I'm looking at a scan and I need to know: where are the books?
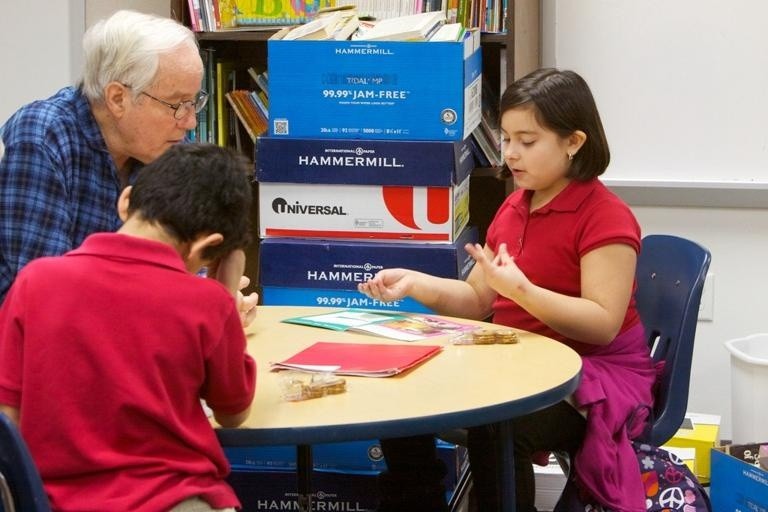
[267,340,445,379]
[187,1,507,169]
[279,306,484,344]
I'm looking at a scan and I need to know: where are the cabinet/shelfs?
[170,1,541,305]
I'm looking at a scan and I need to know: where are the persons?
[0,139,264,512]
[354,66,659,511]
[0,8,206,302]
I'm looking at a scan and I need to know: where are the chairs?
[550,234,710,447]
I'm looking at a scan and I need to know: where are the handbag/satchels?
[585,440,713,512]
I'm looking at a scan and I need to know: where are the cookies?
[301,379,345,397]
[472,328,516,344]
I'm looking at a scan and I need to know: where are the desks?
[199,304,582,512]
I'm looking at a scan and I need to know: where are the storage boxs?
[708,442,766,510]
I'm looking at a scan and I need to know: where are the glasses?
[123,84,209,120]
